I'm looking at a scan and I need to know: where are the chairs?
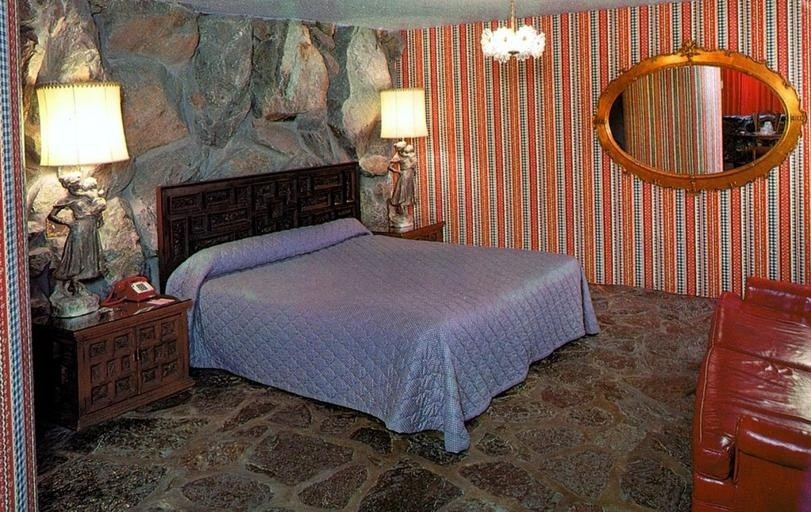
[735,114,776,161]
[763,114,787,143]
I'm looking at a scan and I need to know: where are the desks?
[375,221,447,242]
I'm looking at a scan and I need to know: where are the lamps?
[381,88,430,230]
[480,0,546,66]
[38,84,131,317]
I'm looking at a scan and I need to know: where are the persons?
[69,176,107,229]
[45,170,109,295]
[397,145,417,208]
[387,141,415,216]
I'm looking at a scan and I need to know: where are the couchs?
[692,274,811,512]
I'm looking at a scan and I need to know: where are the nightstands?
[33,297,193,432]
[156,161,600,452]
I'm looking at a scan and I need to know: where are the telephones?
[115,276,156,302]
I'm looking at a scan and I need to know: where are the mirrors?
[593,39,808,197]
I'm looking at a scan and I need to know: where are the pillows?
[198,218,364,276]
